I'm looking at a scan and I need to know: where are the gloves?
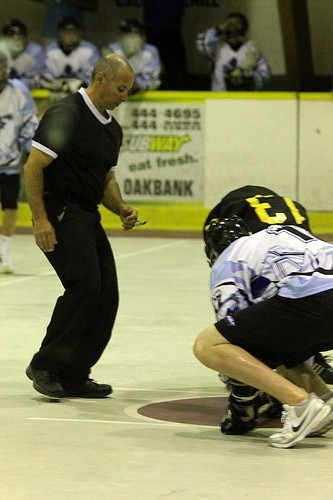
[220,392,284,435]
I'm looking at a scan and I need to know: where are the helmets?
[120,18,142,35]
[202,215,250,267]
[3,19,27,38]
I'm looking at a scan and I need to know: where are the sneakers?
[302,352,333,384]
[267,392,333,448]
[0,253,13,274]
[65,377,111,397]
[26,359,65,398]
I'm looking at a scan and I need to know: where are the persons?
[23,54,137,400]
[1,51,42,277]
[194,185,333,448]
[2,13,271,95]
